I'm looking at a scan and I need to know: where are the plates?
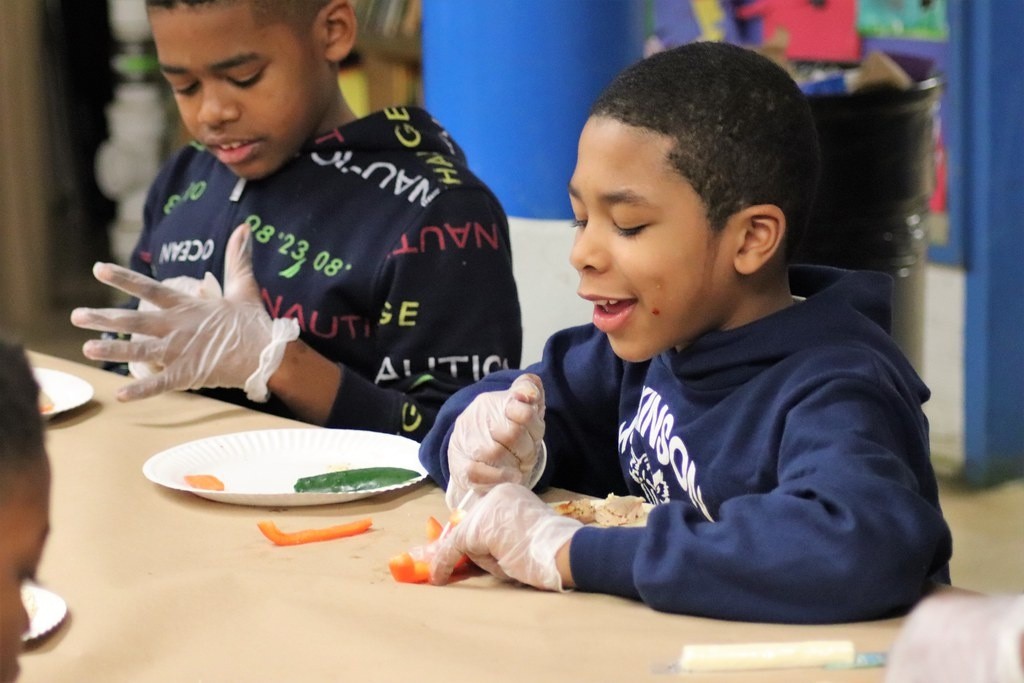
[139,427,431,509]
[14,582,69,644]
[30,368,99,422]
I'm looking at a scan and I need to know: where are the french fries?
[257,520,371,544]
[389,519,469,583]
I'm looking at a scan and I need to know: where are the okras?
[293,467,420,493]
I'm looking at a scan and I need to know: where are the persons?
[0,344,50,683]
[419,41,953,624]
[71,0,522,442]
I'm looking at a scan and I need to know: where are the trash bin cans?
[786,56,944,395]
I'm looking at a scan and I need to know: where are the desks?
[17,347,989,682]
